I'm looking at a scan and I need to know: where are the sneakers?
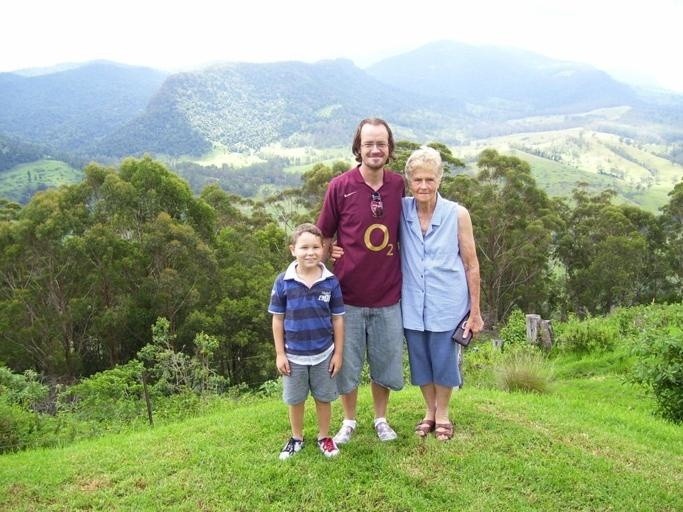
[317,425,355,458]
[373,421,397,442]
[278,437,305,461]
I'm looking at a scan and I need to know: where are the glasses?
[360,142,390,150]
[370,190,384,217]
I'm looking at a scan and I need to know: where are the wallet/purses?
[452,310,474,348]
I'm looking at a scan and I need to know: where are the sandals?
[414,416,455,441]
